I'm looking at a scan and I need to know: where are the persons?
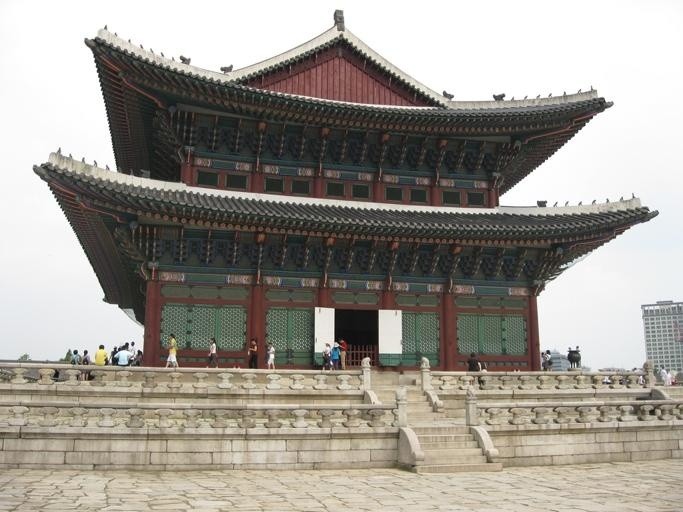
[338,337,348,370]
[265,342,276,369]
[541,352,547,361]
[329,342,340,370]
[466,351,479,371]
[321,343,332,370]
[245,339,259,368]
[164,333,180,369]
[601,366,678,386]
[67,341,144,381]
[206,337,219,368]
[541,349,552,371]
